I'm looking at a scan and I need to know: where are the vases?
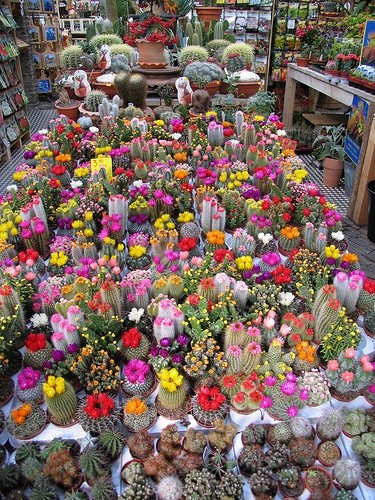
[295,57,311,67]
[92,81,118,99]
[195,7,223,29]
[326,70,339,77]
[134,39,164,64]
[232,81,263,99]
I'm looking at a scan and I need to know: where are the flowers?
[0,0,375,425]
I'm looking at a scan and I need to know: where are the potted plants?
[312,123,346,187]
[80,53,102,83]
[78,88,109,118]
[183,61,223,97]
[55,81,81,122]
[188,90,213,118]
[0,406,375,500]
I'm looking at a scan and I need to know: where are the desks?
[282,62,375,225]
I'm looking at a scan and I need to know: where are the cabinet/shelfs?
[0,0,32,168]
[25,0,65,101]
